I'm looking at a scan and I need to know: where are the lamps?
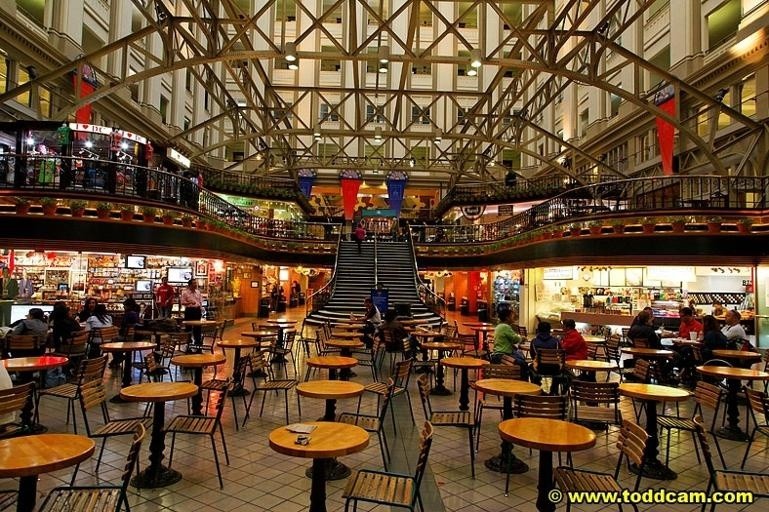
[379,65,388,73]
[288,54,299,70]
[285,42,296,62]
[374,126,383,139]
[379,46,389,64]
[471,49,482,68]
[313,124,321,137]
[466,58,477,76]
[434,128,441,141]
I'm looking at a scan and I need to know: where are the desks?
[260,323,293,364]
[325,339,364,378]
[217,338,259,397]
[136,328,191,350]
[421,341,462,395]
[183,319,217,351]
[440,357,490,427]
[660,337,690,382]
[267,319,297,353]
[100,340,157,404]
[295,380,364,481]
[498,417,597,512]
[0,357,68,435]
[332,332,364,359]
[120,382,199,491]
[618,383,689,481]
[461,322,493,355]
[241,330,278,378]
[306,357,359,423]
[335,323,364,332]
[469,326,495,361]
[620,346,673,403]
[696,365,768,441]
[168,353,226,416]
[0,433,96,512]
[269,421,369,512]
[566,359,618,431]
[712,348,761,405]
[410,331,447,375]
[475,378,541,474]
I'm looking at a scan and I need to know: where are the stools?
[699,469,769,512]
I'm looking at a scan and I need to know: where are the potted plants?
[552,224,563,238]
[570,223,582,236]
[706,218,722,231]
[588,221,602,234]
[671,218,686,231]
[40,197,58,215]
[69,199,88,218]
[161,210,177,225]
[13,195,30,215]
[195,216,205,229]
[119,206,135,221]
[612,221,625,234]
[140,206,157,223]
[95,201,112,218]
[181,214,192,227]
[736,218,754,234]
[640,218,657,233]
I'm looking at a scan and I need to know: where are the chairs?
[32,353,108,434]
[415,372,476,477]
[139,338,176,383]
[454,320,475,356]
[338,377,395,471]
[402,337,436,389]
[216,320,236,357]
[380,327,406,376]
[456,326,481,358]
[88,326,111,358]
[4,334,39,358]
[622,359,649,422]
[502,393,573,498]
[197,354,250,431]
[605,343,621,382]
[355,357,415,433]
[474,364,519,452]
[603,334,630,383]
[52,331,89,378]
[461,337,483,381]
[312,328,341,376]
[68,378,154,495]
[528,348,566,395]
[470,326,495,355]
[164,388,230,489]
[341,420,434,511]
[634,338,662,384]
[99,327,125,381]
[622,328,634,350]
[354,336,382,382]
[690,342,718,385]
[242,352,302,428]
[0,381,35,442]
[657,381,727,471]
[38,423,145,512]
[580,333,608,360]
[552,419,650,512]
[587,344,607,363]
[565,379,633,470]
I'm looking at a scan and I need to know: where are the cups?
[689,332,697,340]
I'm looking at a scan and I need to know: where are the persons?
[108,298,139,369]
[13,308,49,358]
[355,224,364,253]
[352,297,381,349]
[494,309,530,382]
[644,306,654,321]
[628,311,680,385]
[557,319,587,395]
[700,315,728,383]
[1,360,17,425]
[50,301,81,363]
[180,279,202,354]
[379,309,422,353]
[293,280,301,306]
[179,169,203,212]
[86,304,113,358]
[713,303,728,317]
[675,308,704,337]
[80,297,97,323]
[721,310,748,339]
[530,322,561,395]
[156,277,175,317]
